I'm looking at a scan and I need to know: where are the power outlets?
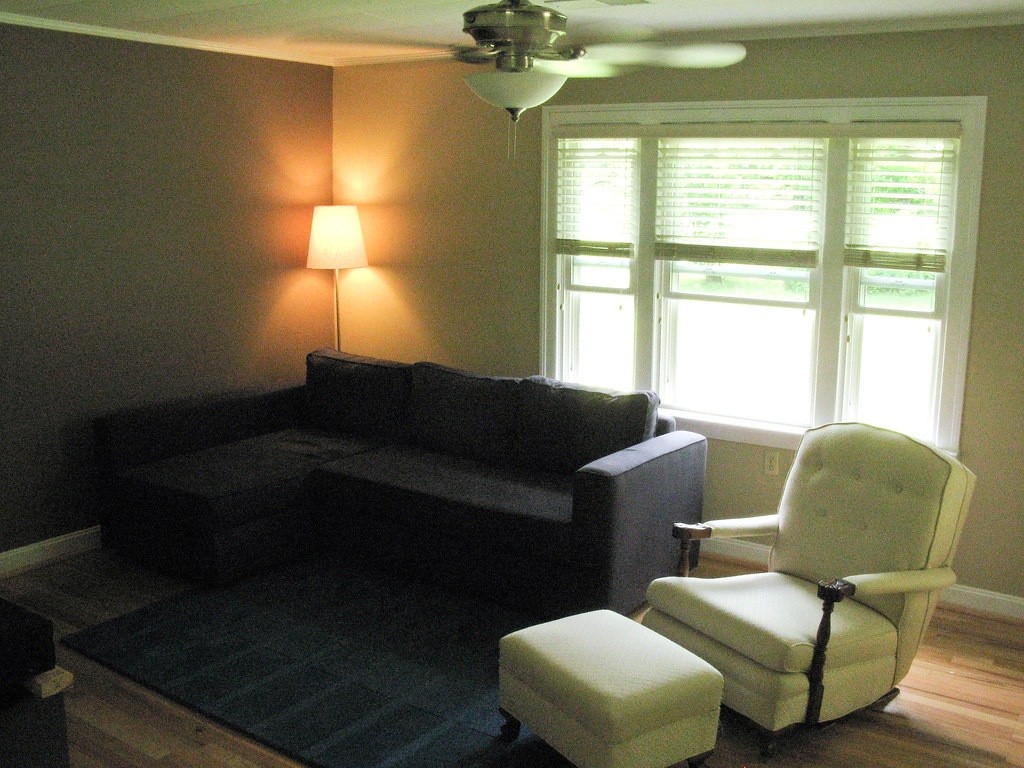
[764,451,779,476]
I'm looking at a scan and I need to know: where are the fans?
[281,0,747,78]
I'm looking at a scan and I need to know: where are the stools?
[497,609,725,768]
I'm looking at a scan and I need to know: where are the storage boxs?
[30,665,74,698]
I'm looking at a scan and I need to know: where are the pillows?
[307,348,415,442]
[516,374,659,473]
[408,361,522,467]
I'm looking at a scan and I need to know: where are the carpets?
[62,549,574,768]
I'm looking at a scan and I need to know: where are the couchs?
[642,421,977,763]
[90,380,707,616]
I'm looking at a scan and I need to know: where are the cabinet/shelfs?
[0,596,69,768]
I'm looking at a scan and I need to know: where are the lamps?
[306,205,369,351]
[461,70,568,123]
